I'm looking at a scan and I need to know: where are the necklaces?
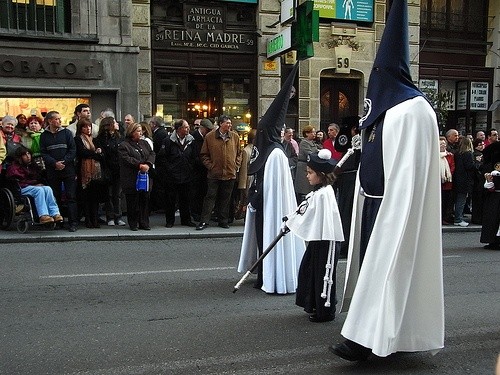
[280,149,345,322]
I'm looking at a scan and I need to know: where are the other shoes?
[70,225,78,232]
[108,219,126,226]
[41,215,64,223]
[304,308,335,322]
[484,244,500,251]
[454,220,469,226]
[132,226,151,230]
[166,223,173,228]
[328,341,366,359]
[182,221,196,227]
[85,216,100,228]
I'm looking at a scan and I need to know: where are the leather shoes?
[196,223,206,230]
[222,224,229,228]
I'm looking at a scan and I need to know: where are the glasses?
[492,133,499,136]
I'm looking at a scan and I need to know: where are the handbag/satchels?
[234,190,247,221]
[137,169,150,191]
[34,157,47,171]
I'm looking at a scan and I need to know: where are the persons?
[329,50,444,362]
[281,123,361,223]
[480,141,500,246]
[0,104,257,231]
[336,134,361,259]
[440,128,500,227]
[237,110,307,294]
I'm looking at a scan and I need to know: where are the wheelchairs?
[0,188,65,234]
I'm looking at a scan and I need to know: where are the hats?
[306,149,333,171]
[201,119,214,130]
